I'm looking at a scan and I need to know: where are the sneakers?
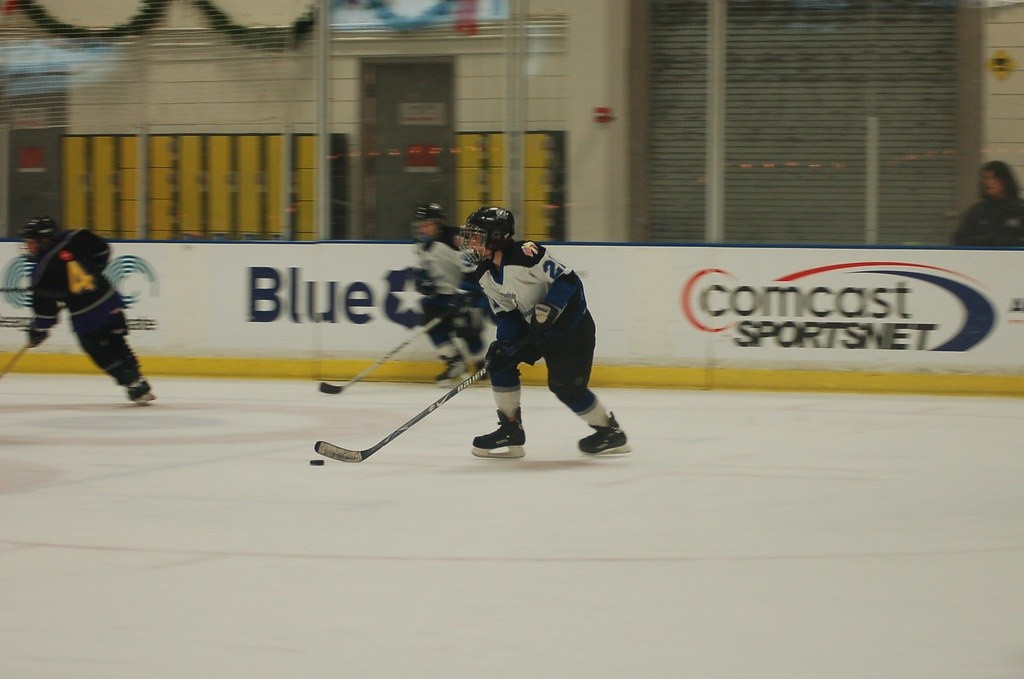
[472,402,525,458]
[578,410,633,456]
[128,378,156,403]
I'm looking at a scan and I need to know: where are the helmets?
[408,202,448,244]
[459,207,516,265]
[15,218,59,262]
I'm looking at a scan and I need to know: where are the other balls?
[310,459,325,466]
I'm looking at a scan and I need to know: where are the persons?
[412,203,488,387]
[954,161,1024,247]
[18,216,154,402]
[457,206,631,459]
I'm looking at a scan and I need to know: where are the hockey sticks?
[318,309,456,395]
[312,333,534,465]
[0,306,64,380]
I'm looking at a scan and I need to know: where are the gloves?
[484,341,517,375]
[447,288,474,316]
[416,277,434,294]
[28,322,49,345]
[528,303,561,347]
